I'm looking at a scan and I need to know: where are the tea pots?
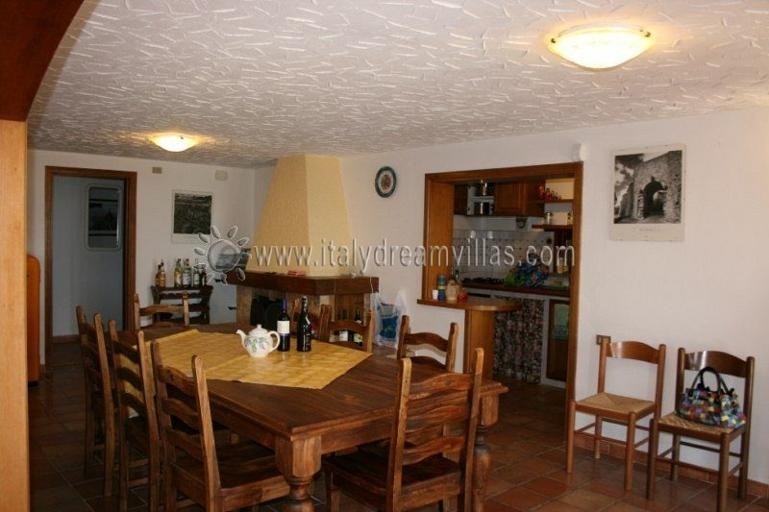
[236,324,281,359]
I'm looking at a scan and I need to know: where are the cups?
[545,211,552,224]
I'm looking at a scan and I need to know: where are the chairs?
[644,347,756,512]
[565,338,667,491]
[76,292,511,512]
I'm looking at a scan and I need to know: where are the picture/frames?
[83,183,124,252]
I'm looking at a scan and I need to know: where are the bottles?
[296,296,312,352]
[275,294,292,352]
[352,307,364,346]
[432,269,468,304]
[156,255,207,288]
[337,309,348,343]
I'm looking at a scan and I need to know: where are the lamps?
[147,131,198,153]
[548,21,656,70]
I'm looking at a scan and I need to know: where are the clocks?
[375,166,397,199]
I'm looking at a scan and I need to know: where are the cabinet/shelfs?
[530,198,573,232]
[494,179,546,218]
[454,184,494,217]
[151,284,214,326]
